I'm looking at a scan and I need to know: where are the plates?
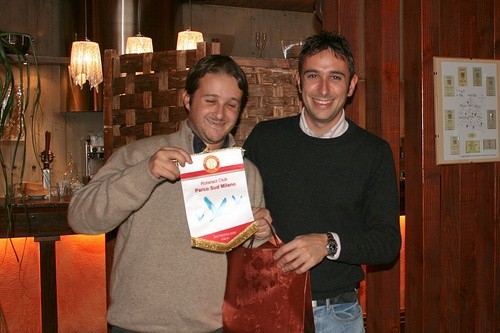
[29,195,46,199]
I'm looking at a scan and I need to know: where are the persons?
[67,54,274,333]
[242,31,402,333]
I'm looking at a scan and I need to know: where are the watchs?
[325,232,338,256]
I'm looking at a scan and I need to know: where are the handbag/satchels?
[222,219,315,333]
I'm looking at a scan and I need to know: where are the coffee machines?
[80,132,104,184]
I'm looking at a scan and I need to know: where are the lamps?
[71,0,103,94]
[126,0,154,55]
[176,0,205,51]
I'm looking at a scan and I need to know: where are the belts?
[312,288,357,307]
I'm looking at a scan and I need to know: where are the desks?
[0,196,118,309]
